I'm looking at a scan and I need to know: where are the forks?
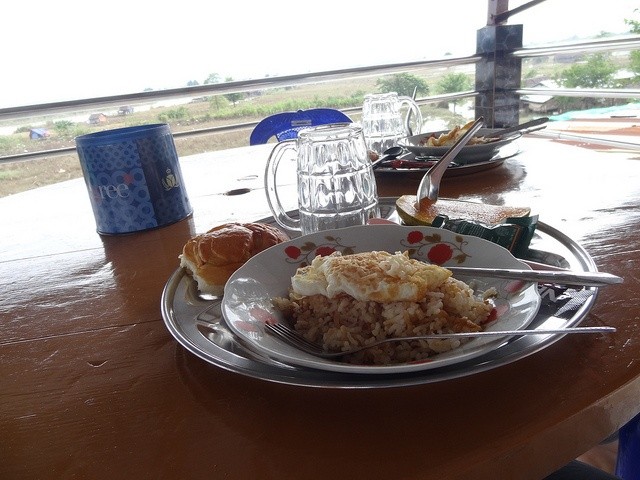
[264,320,618,359]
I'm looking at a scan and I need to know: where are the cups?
[361,93,423,156]
[265,124,381,239]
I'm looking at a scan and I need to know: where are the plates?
[396,127,521,163]
[160,196,601,390]
[221,225,541,374]
[374,148,519,179]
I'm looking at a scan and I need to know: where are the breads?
[178,222,289,296]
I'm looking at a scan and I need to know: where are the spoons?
[417,116,485,208]
[373,147,404,168]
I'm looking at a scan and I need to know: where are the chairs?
[249,109,352,145]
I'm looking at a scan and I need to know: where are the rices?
[272,276,498,364]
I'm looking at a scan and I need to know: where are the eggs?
[291,250,454,302]
